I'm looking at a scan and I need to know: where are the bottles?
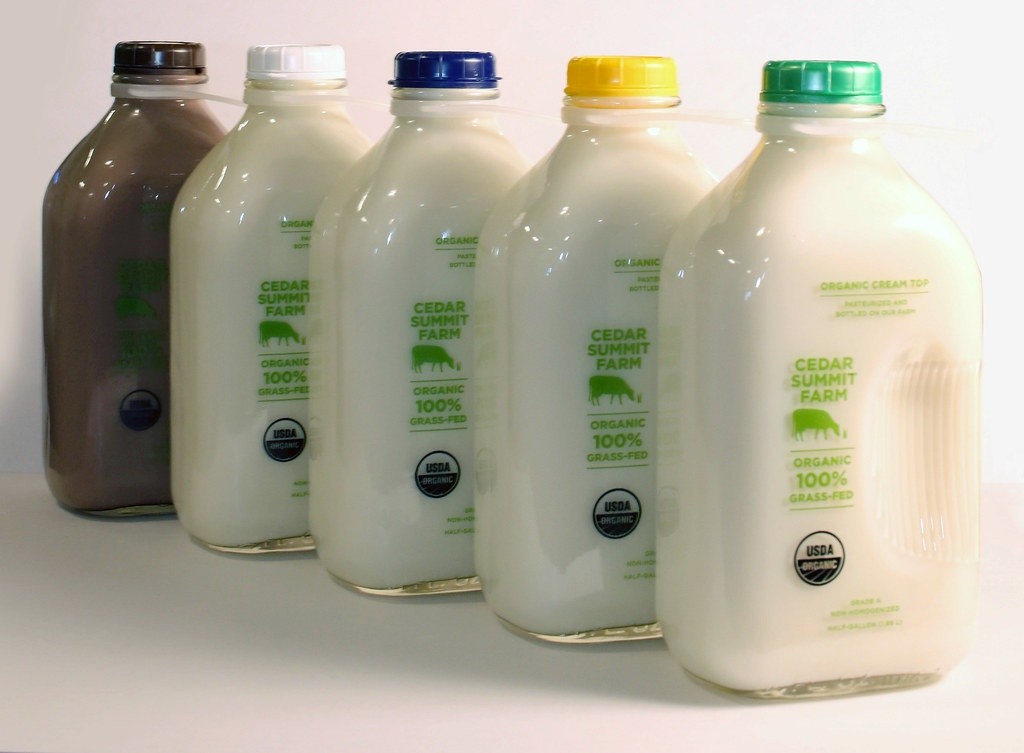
[304,48,536,596]
[466,51,725,646]
[39,39,235,521]
[168,42,377,557]
[651,59,984,702]
